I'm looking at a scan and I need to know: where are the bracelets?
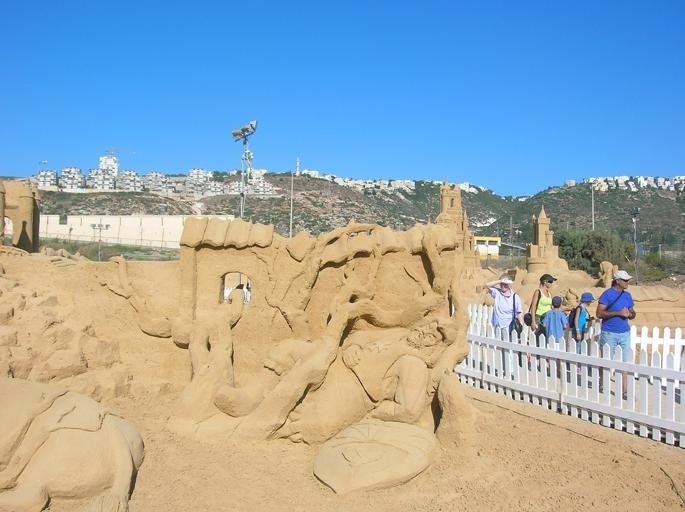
[576,327,580,331]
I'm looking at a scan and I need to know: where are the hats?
[614,271,634,280]
[540,274,557,281]
[580,293,595,302]
[552,296,561,306]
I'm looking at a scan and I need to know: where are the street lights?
[589,182,598,231]
[92,224,108,261]
[631,207,640,256]
[233,120,257,220]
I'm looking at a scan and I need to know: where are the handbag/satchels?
[569,306,583,328]
[509,318,522,338]
[524,313,541,326]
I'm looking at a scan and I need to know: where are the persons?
[211,313,458,446]
[542,296,569,378]
[485,273,525,344]
[571,291,596,376]
[597,270,637,400]
[529,272,577,373]
